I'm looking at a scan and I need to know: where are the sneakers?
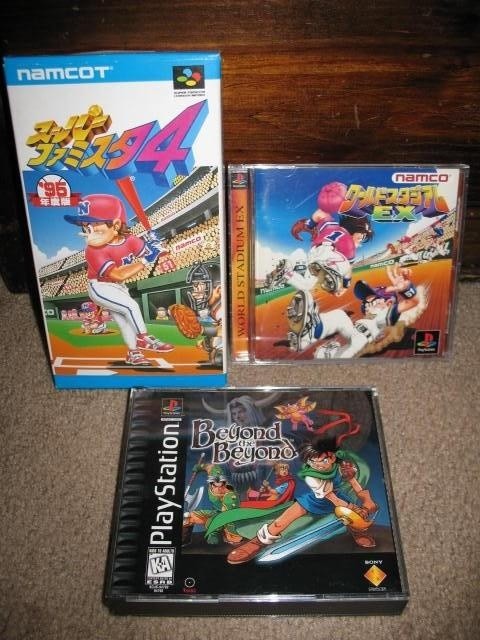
[124,350,153,366]
[308,262,339,293]
[136,334,175,352]
[286,290,316,354]
[262,259,289,292]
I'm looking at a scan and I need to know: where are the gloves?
[142,239,167,265]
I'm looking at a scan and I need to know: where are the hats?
[64,194,126,225]
[318,181,367,218]
[79,302,98,312]
[354,280,397,316]
